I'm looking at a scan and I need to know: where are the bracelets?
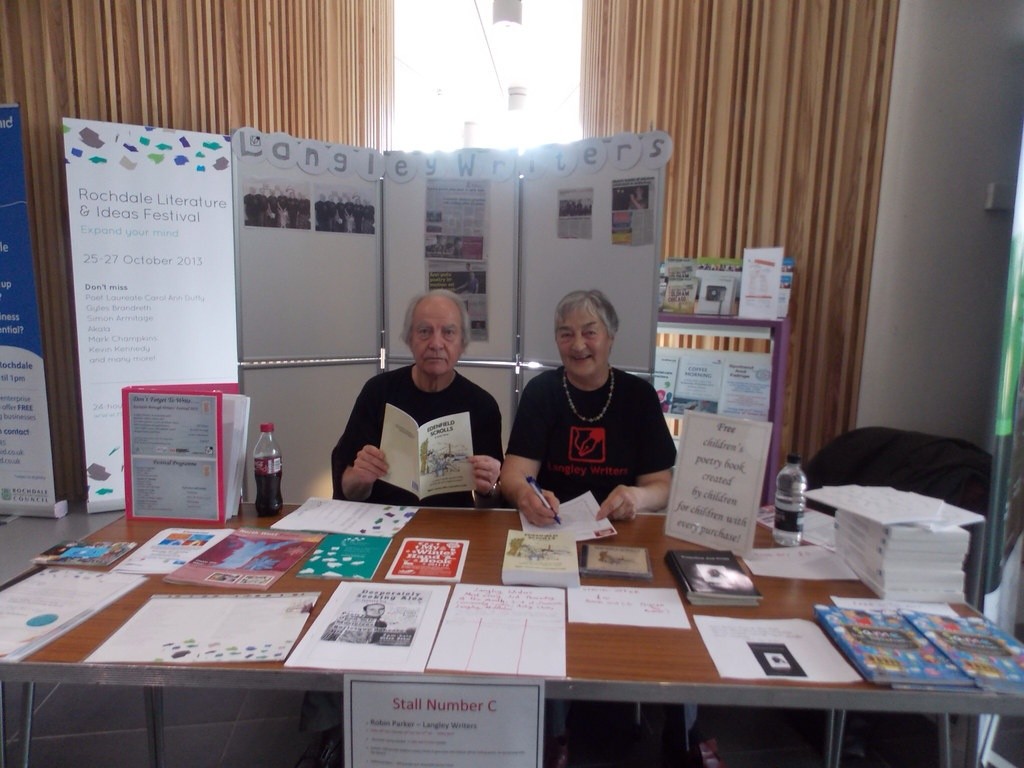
[489,483,497,497]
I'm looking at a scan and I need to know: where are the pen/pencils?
[525,475,564,525]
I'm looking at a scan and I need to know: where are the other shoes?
[603,712,650,759]
[662,720,730,768]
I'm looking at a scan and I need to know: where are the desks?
[0,501,1024,768]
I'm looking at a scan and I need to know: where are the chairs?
[805,427,1004,596]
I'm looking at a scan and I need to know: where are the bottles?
[772,453,807,547]
[253,423,283,517]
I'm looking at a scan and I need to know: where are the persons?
[330,290,504,508]
[500,289,676,526]
[429,235,464,258]
[244,193,375,234]
[628,187,647,210]
[455,263,478,294]
[559,197,592,216]
[362,603,387,643]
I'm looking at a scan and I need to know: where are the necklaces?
[424,371,456,391]
[563,365,614,422]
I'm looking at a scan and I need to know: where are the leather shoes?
[546,728,570,768]
[316,725,343,768]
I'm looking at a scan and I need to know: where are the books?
[32,540,138,567]
[811,596,1024,693]
[385,538,471,584]
[294,532,393,580]
[502,529,581,588]
[161,520,327,592]
[377,404,477,500]
[666,549,763,608]
[832,509,970,603]
